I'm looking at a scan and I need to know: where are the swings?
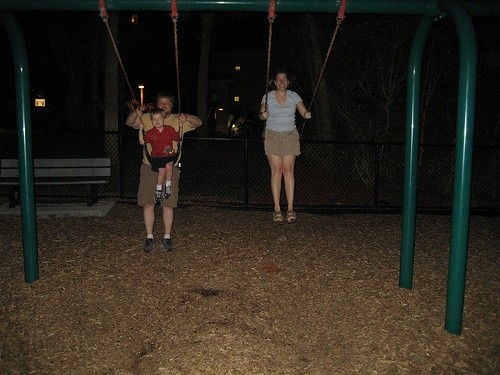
[259,0,346,142]
[97,0,185,173]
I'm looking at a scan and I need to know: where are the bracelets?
[184,113,188,121]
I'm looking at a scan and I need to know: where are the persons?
[259,69,312,224]
[139,108,184,205]
[126,91,202,252]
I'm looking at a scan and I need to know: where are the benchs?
[0,158,111,208]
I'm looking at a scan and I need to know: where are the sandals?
[286,210,296,223]
[273,210,285,224]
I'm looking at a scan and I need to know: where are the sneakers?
[143,238,155,253]
[160,238,172,251]
[154,189,164,203]
[164,184,173,199]
